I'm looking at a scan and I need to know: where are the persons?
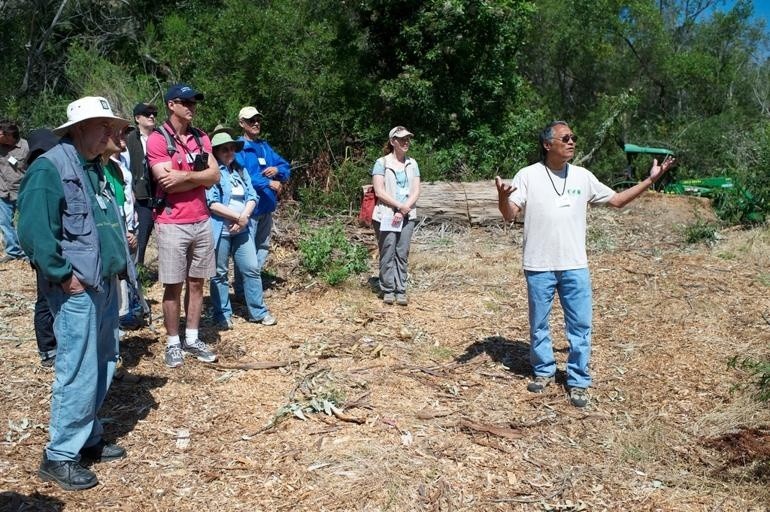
[494,119,676,408]
[145,85,222,367]
[33,266,58,367]
[231,105,291,306]
[371,125,421,307]
[2,100,236,333]
[204,132,277,332]
[16,95,133,494]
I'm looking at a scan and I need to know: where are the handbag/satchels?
[358,157,387,229]
[117,262,152,330]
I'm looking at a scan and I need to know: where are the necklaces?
[543,160,568,197]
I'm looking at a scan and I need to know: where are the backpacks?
[144,122,208,214]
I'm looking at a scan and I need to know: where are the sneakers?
[165,311,277,369]
[564,382,588,407]
[79,438,126,463]
[383,293,394,305]
[527,374,556,392]
[39,356,56,373]
[38,450,98,491]
[395,293,408,306]
[114,356,139,384]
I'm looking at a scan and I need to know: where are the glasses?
[550,135,579,143]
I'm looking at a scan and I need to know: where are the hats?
[388,126,415,139]
[24,85,265,163]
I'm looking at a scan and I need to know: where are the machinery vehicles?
[606,145,736,208]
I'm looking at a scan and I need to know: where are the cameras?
[193,153,208,171]
